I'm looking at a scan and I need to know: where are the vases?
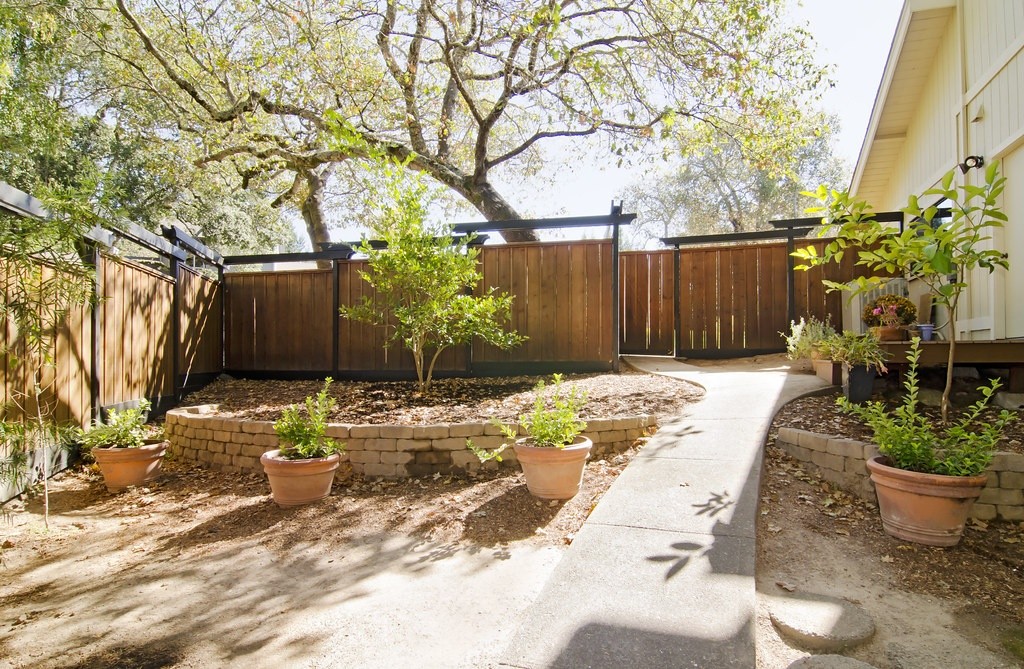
[915,325,934,341]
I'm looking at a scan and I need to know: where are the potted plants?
[861,294,916,341]
[463,372,593,499]
[77,397,168,493]
[260,377,346,509]
[777,315,838,372]
[828,330,894,408]
[835,336,1023,548]
[811,337,842,386]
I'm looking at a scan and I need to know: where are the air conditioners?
[841,277,906,337]
